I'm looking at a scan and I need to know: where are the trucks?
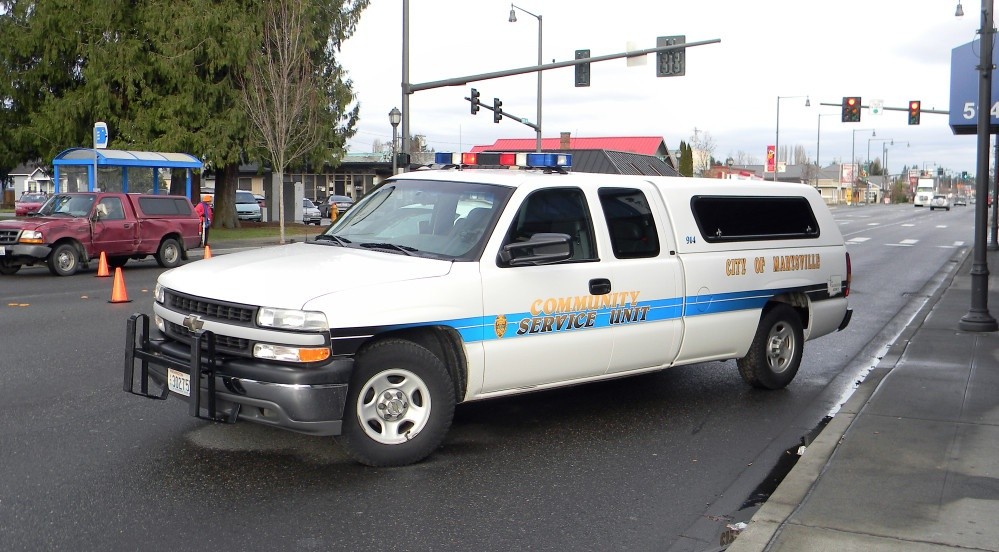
[913,178,939,193]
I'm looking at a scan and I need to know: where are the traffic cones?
[107,267,133,303]
[94,251,113,277]
[202,245,211,260]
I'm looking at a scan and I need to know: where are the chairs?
[454,206,491,235]
[553,220,582,259]
[611,223,646,256]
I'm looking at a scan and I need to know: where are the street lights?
[727,157,734,179]
[775,96,810,180]
[851,129,876,201]
[388,107,402,176]
[922,161,936,173]
[867,137,910,205]
[509,3,542,152]
[676,149,682,176]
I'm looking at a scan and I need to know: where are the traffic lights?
[842,98,861,122]
[471,88,480,114]
[962,172,967,182]
[494,98,502,123]
[908,100,920,125]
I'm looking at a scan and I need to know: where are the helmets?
[203,195,213,202]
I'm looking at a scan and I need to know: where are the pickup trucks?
[122,152,853,468]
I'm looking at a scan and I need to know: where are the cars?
[16,191,50,217]
[146,188,265,222]
[914,192,950,211]
[954,192,994,208]
[302,195,354,226]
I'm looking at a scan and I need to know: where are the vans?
[0,192,203,275]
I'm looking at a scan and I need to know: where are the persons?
[194,195,212,247]
[100,198,120,218]
